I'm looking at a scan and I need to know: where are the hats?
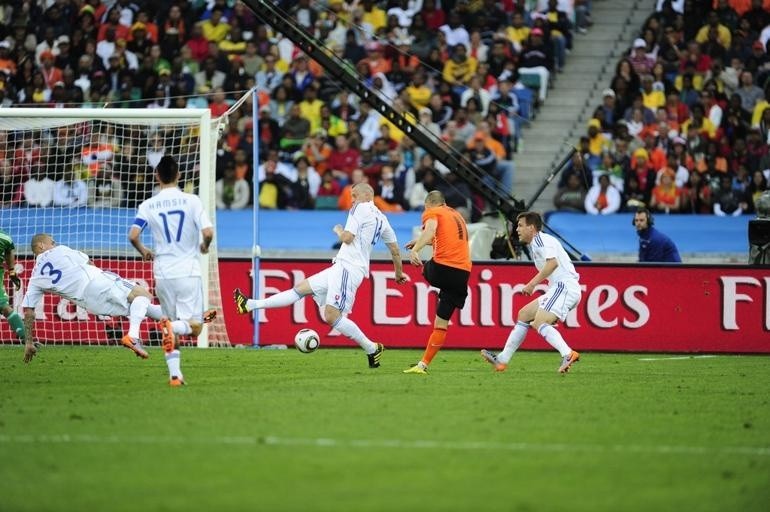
[133,21,147,30]
[603,88,615,97]
[80,4,94,13]
[589,118,601,127]
[58,35,69,46]
[672,137,686,145]
[40,52,52,63]
[635,147,649,161]
[634,38,646,48]
[159,69,171,76]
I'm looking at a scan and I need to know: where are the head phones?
[632,207,654,227]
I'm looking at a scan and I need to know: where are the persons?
[748,189,770,266]
[0,231,44,348]
[128,154,216,388]
[480,211,583,373]
[1,0,589,227]
[554,0,770,218]
[402,189,473,374]
[232,180,411,370]
[632,206,683,262]
[20,233,218,364]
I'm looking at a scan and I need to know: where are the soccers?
[294,329,320,353]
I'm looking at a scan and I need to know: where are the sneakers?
[233,288,249,313]
[559,350,579,372]
[160,319,175,352]
[367,343,384,367]
[480,349,507,370]
[204,310,216,323]
[122,334,148,358]
[171,376,186,385]
[403,365,426,373]
